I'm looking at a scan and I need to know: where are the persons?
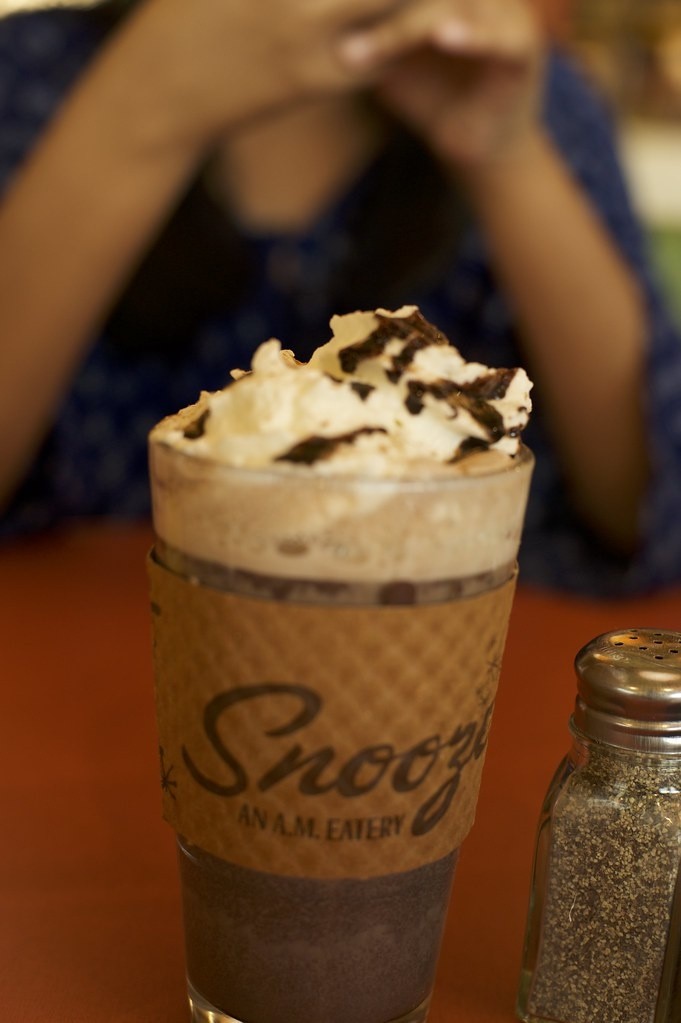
[0,0,681,600]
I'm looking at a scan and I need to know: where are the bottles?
[517,628,681,1023]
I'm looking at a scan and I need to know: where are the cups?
[150,422,533,1023]
